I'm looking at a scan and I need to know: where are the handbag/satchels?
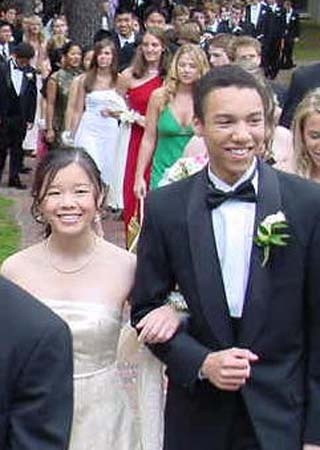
[127,195,143,254]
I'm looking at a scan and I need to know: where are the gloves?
[61,131,76,149]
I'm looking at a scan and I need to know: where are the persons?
[130,64,320,450]
[0,0,320,223]
[0,274,74,450]
[0,146,188,450]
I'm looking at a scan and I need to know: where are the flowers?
[25,72,36,82]
[253,211,293,266]
[157,154,205,188]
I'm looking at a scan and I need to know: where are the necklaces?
[46,230,97,274]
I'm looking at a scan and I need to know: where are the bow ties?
[205,163,258,210]
[12,60,24,71]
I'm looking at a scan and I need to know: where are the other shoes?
[9,178,27,190]
[18,165,33,172]
[26,150,36,158]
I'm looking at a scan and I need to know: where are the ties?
[2,48,7,59]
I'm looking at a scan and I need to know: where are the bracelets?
[169,293,188,312]
